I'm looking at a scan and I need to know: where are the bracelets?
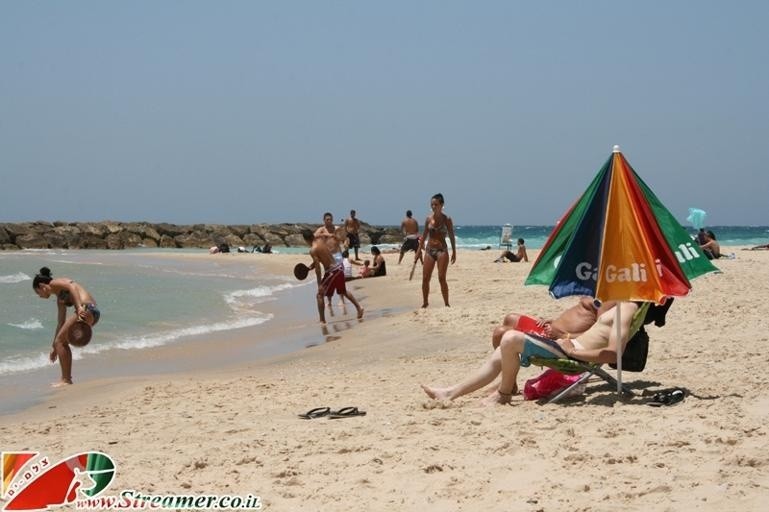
[567,347,575,357]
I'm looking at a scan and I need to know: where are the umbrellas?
[523,144,721,399]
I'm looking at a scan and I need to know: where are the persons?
[341,250,364,281]
[208,242,273,256]
[418,300,642,409]
[312,213,351,316]
[698,227,707,243]
[303,229,366,323]
[491,295,616,395]
[361,246,386,277]
[494,237,529,263]
[32,265,101,390]
[343,208,362,260]
[413,193,457,308]
[359,260,370,277]
[698,230,721,260]
[397,209,424,264]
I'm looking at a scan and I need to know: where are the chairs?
[528,298,652,405]
